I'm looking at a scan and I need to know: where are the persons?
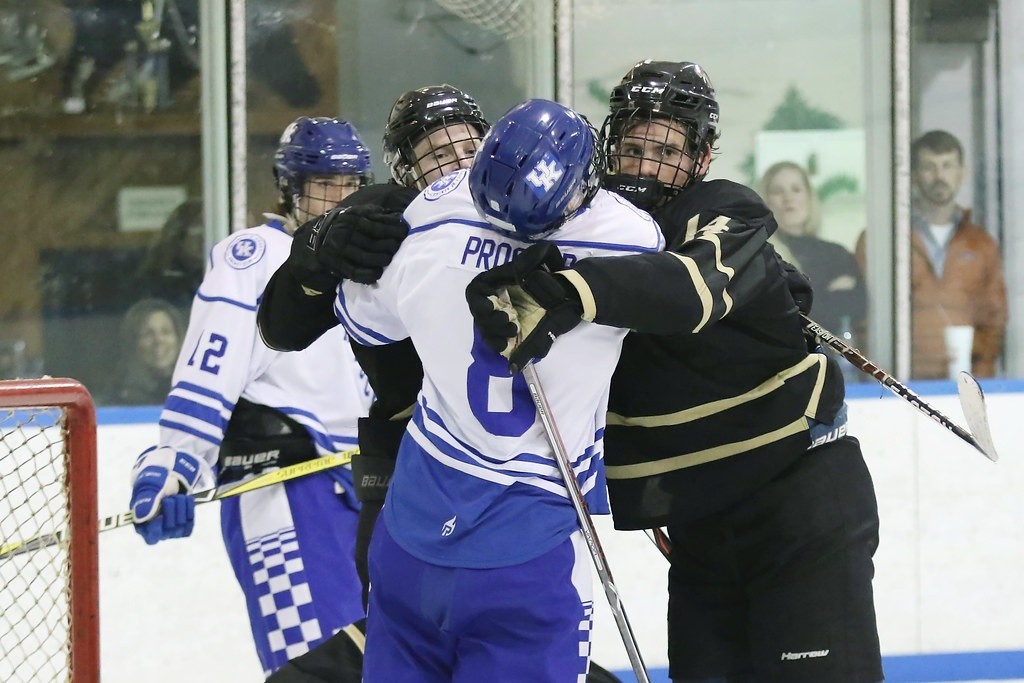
[127,115,377,683]
[314,59,885,683]
[256,84,490,614]
[334,99,666,683]
[850,130,1008,386]
[59,0,200,114]
[101,297,187,406]
[761,160,866,339]
[130,202,205,304]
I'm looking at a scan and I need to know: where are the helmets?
[272,117,376,230]
[469,99,607,242]
[382,83,491,194]
[595,60,721,215]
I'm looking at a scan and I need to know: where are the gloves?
[465,241,584,378]
[130,445,218,545]
[290,204,410,292]
